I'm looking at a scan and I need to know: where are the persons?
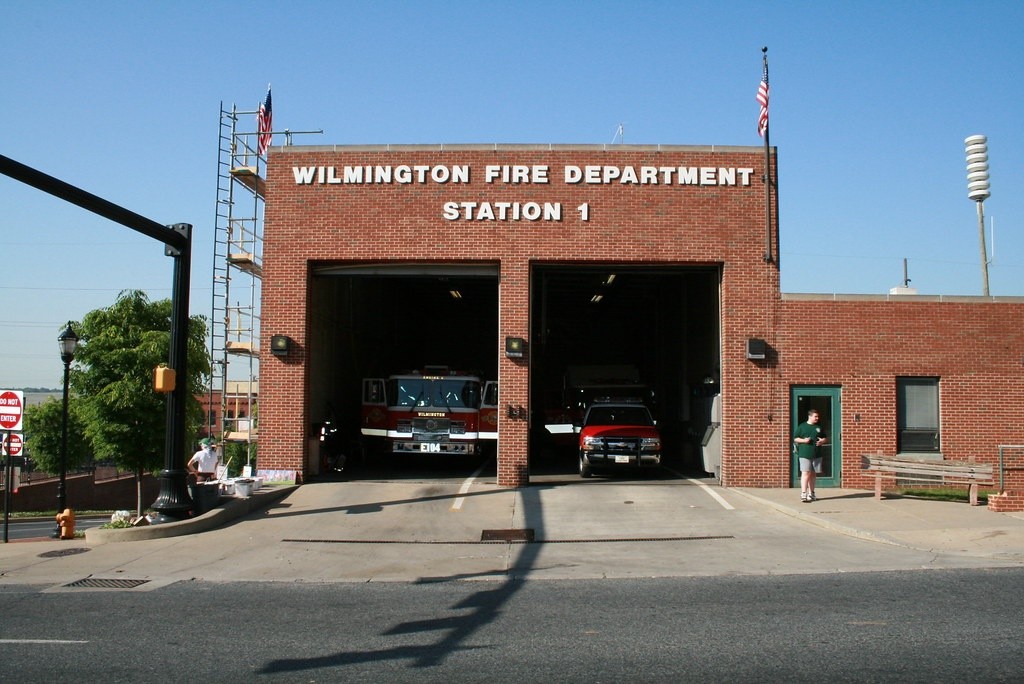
[188,438,219,515]
[794,410,827,502]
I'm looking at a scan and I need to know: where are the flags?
[256,87,273,158]
[755,55,771,137]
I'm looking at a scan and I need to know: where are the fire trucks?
[359,362,499,457]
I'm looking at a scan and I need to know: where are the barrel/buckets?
[215,476,264,495]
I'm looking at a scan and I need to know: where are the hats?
[198,438,211,446]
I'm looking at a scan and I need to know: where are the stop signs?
[2,432,24,457]
[0,389,24,432]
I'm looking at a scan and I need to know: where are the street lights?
[49,319,78,538]
[965,133,994,296]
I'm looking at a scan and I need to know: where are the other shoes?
[807,491,816,501]
[800,492,807,502]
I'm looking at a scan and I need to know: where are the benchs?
[859,451,995,506]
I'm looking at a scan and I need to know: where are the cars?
[578,395,663,478]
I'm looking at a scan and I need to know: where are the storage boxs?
[208,476,264,495]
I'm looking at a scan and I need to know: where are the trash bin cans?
[189,484,220,517]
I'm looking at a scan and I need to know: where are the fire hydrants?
[55,508,76,540]
